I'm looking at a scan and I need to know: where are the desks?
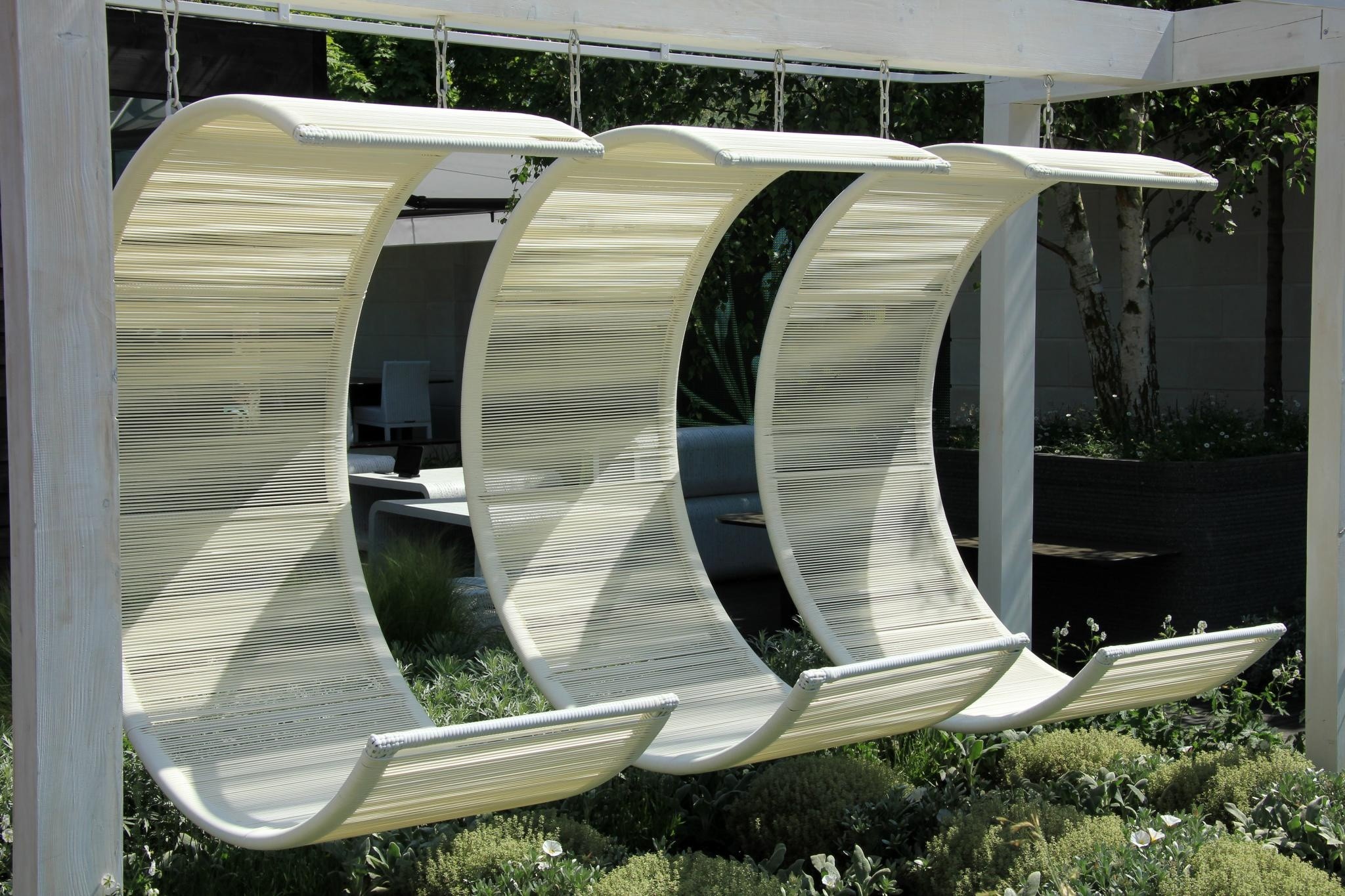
[951,530,1187,680]
[365,496,486,577]
[716,511,803,635]
[344,372,455,444]
[350,465,471,552]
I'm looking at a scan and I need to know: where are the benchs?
[345,439,462,471]
[670,419,782,582]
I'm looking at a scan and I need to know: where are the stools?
[451,573,497,637]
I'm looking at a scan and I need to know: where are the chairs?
[353,358,437,443]
